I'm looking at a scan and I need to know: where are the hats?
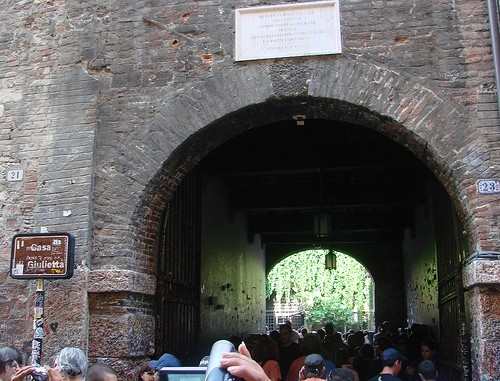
[382,348,407,360]
[149,353,181,371]
[304,354,326,369]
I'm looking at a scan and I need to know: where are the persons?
[0,320,443,381]
